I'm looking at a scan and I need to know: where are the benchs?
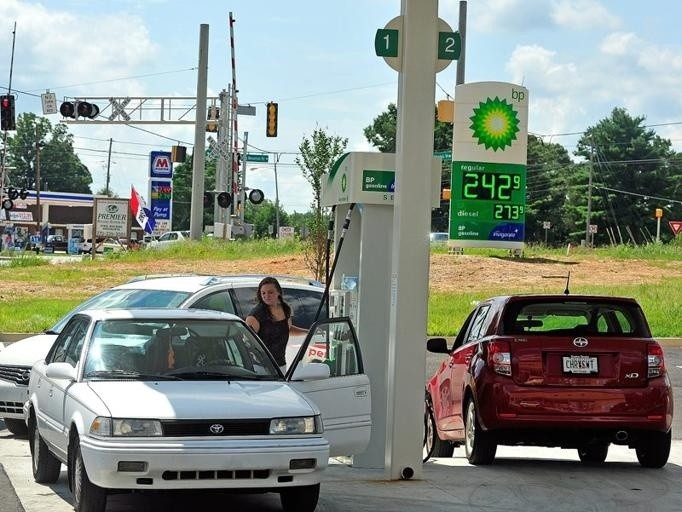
[77,336,186,372]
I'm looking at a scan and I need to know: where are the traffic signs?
[239,154,269,162]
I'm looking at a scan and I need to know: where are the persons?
[245,276,293,376]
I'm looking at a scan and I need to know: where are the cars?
[21,310,374,512]
[429,232,449,243]
[29,230,191,254]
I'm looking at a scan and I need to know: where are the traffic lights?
[266,103,278,137]
[217,192,230,207]
[1,95,16,130]
[78,102,93,116]
[61,103,73,116]
[173,146,186,162]
[206,107,221,133]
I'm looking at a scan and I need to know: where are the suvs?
[0,275,332,434]
[424,271,672,470]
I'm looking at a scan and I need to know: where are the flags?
[129,188,157,236]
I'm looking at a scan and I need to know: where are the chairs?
[183,335,229,368]
